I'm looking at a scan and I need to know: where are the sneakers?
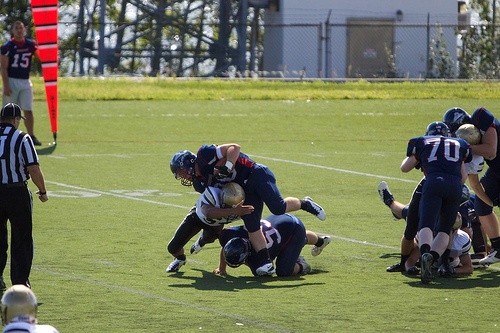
[377,181,395,205]
[479,250,500,264]
[303,195,326,221]
[386,262,405,272]
[166,253,187,273]
[30,135,42,146]
[421,253,433,285]
[255,262,276,277]
[311,235,331,257]
[297,255,313,275]
[190,233,203,255]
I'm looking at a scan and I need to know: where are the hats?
[0,102,27,121]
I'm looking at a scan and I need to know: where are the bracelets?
[36,191,46,195]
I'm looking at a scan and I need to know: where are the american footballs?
[215,167,238,183]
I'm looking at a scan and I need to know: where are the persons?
[377,107,500,285]
[165,143,332,279]
[0,103,49,289]
[0,285,60,333]
[0,21,42,146]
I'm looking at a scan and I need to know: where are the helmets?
[424,121,452,138]
[454,123,481,145]
[222,236,253,268]
[451,212,462,229]
[220,182,246,209]
[170,149,197,186]
[442,107,471,134]
[446,228,472,257]
